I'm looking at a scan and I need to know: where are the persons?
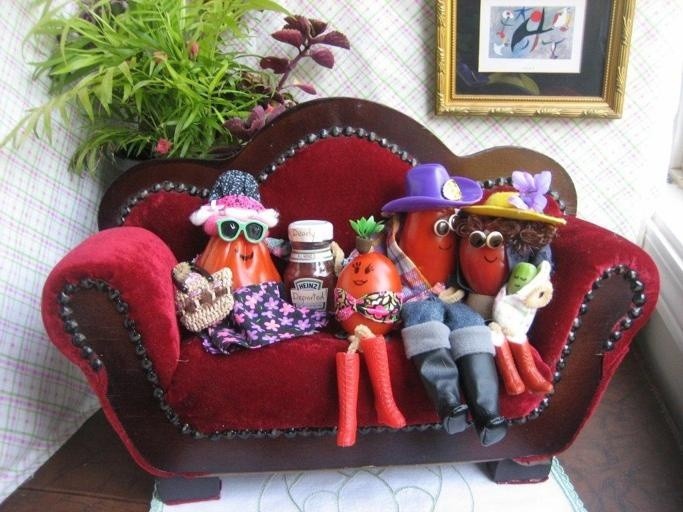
[173,162,568,448]
